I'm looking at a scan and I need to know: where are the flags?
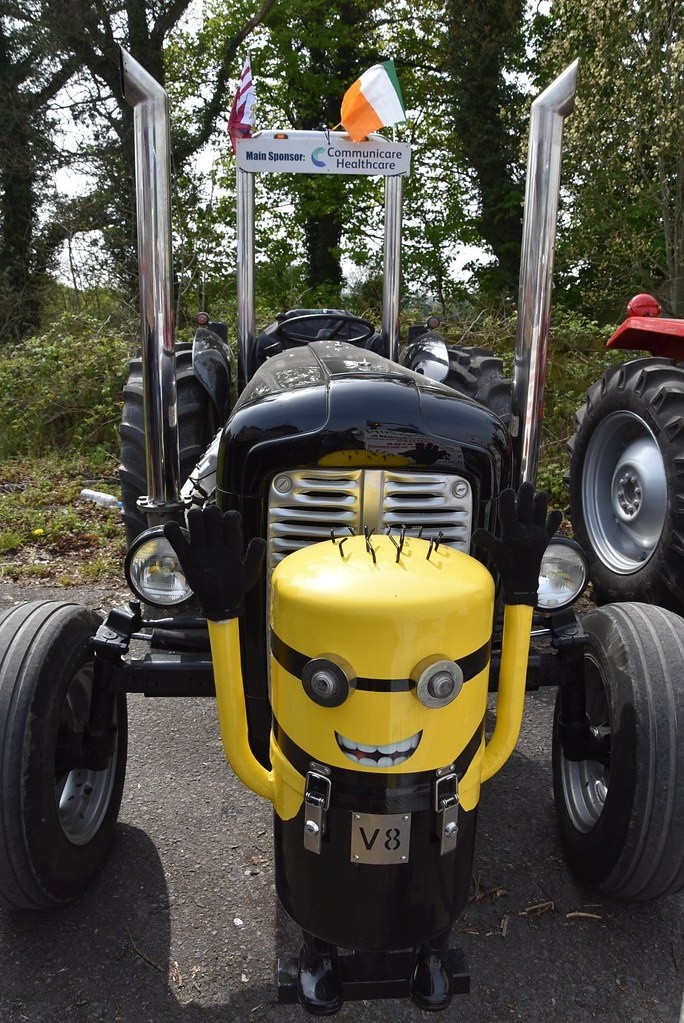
[225,56,255,154]
[340,61,406,142]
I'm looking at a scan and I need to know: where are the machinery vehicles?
[0,44,684,1017]
[561,292,684,607]
